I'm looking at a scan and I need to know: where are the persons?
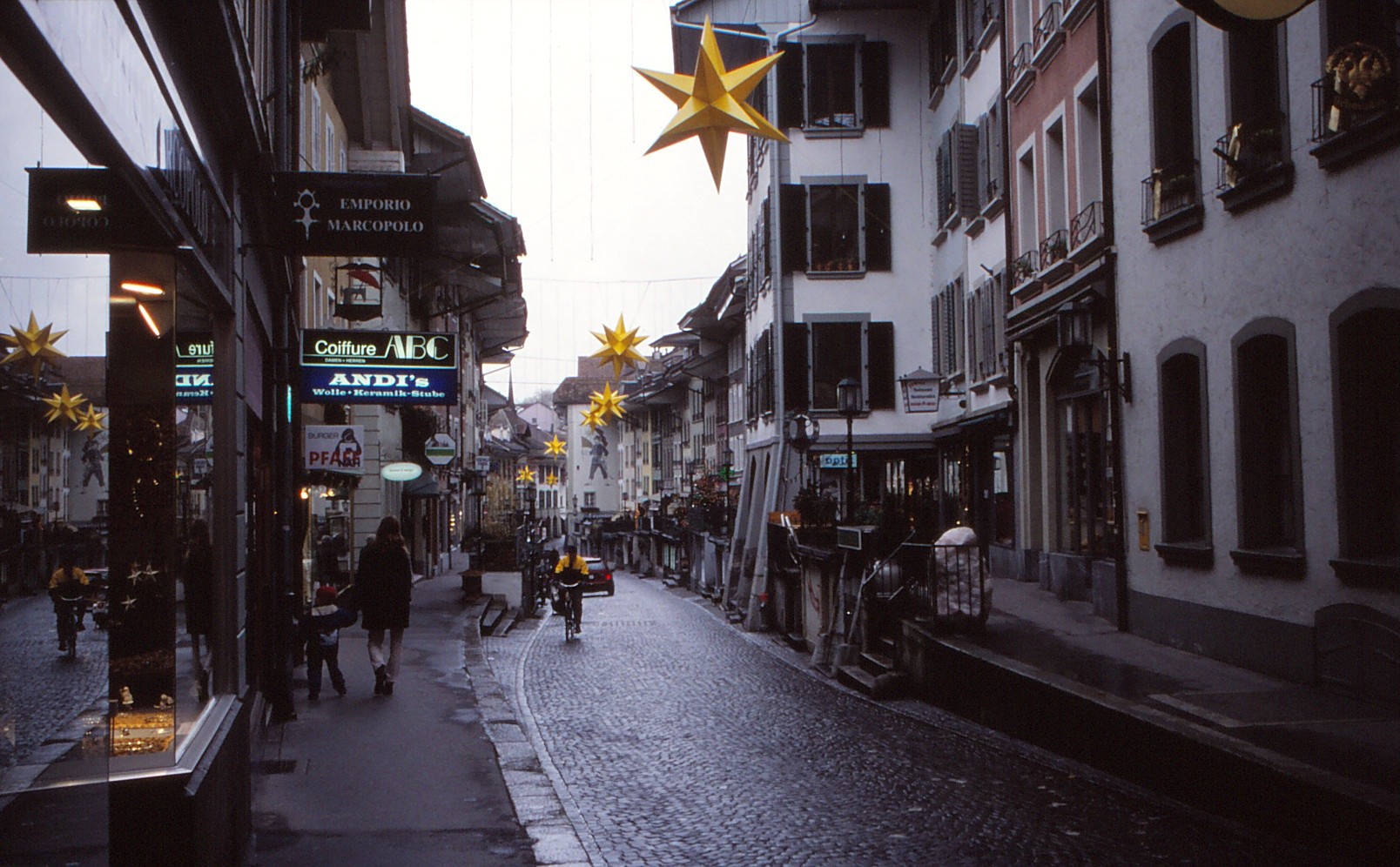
[315,535,338,587]
[554,545,589,633]
[176,516,216,691]
[359,536,375,570]
[299,586,359,702]
[356,516,412,695]
[48,559,90,652]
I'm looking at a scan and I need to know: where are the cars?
[581,557,615,596]
[81,565,109,605]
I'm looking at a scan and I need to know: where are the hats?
[366,534,376,541]
[567,545,578,553]
[322,535,332,542]
[316,586,336,600]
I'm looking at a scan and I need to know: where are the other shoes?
[309,687,319,699]
[575,627,581,632]
[334,681,347,696]
[374,664,386,681]
[383,681,395,694]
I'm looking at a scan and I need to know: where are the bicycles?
[557,580,582,643]
[56,595,85,658]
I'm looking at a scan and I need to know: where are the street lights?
[685,459,698,512]
[523,485,537,595]
[721,446,736,534]
[836,376,864,527]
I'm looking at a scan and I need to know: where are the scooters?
[91,595,111,631]
